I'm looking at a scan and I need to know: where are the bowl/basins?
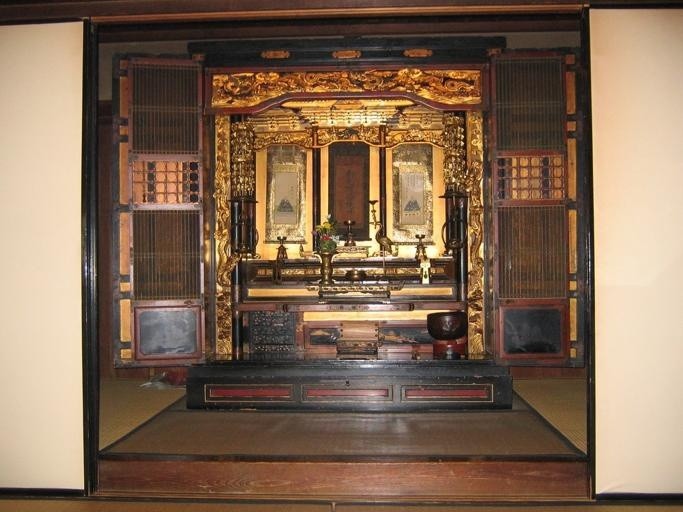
[426,312,466,341]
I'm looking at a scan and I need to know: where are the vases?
[315,252,337,285]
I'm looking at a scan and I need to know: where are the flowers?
[311,220,337,252]
